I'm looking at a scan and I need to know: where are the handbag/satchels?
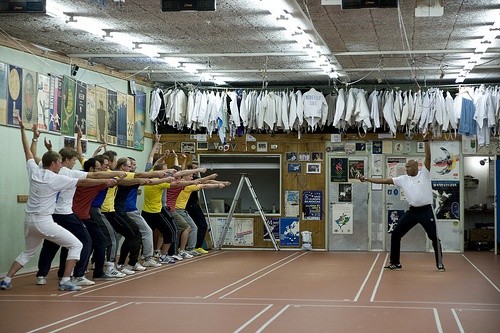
[300,231,312,250]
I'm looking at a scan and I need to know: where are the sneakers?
[93,274,114,281]
[384,264,403,271]
[0,280,12,289]
[436,265,446,272]
[70,262,147,277]
[36,276,47,285]
[59,280,82,291]
[143,259,162,267]
[154,248,208,263]
[72,276,96,286]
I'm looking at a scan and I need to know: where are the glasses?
[104,164,109,166]
[94,167,102,171]
[126,165,131,168]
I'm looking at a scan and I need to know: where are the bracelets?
[423,140,428,142]
[32,138,38,142]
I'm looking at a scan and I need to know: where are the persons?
[0,115,232,291]
[359,128,446,272]
